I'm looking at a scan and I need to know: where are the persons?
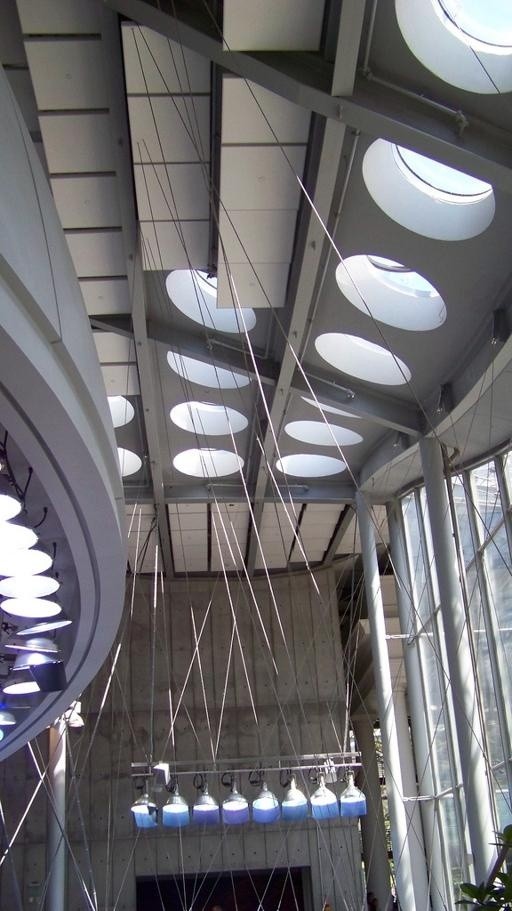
[367,892,378,911]
[324,904,331,911]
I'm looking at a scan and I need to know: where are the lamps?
[130,752,368,828]
[0,428,75,742]
